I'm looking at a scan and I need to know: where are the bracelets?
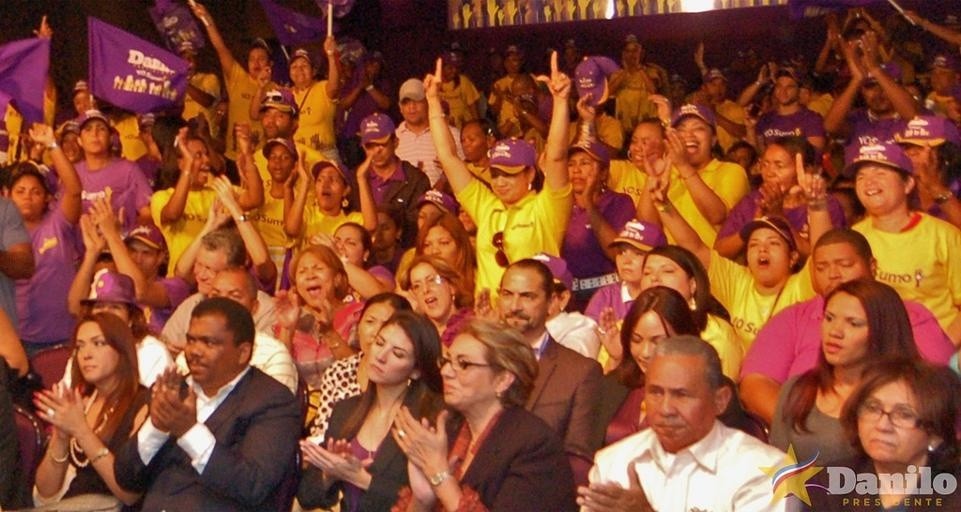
[427,469,451,485]
[88,446,112,461]
[46,449,70,464]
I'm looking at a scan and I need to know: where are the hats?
[775,67,802,84]
[567,140,610,165]
[895,113,961,149]
[739,216,797,247]
[396,78,425,101]
[529,253,572,289]
[608,217,669,254]
[119,224,166,251]
[480,138,536,175]
[436,33,642,107]
[59,109,111,136]
[839,143,912,180]
[701,66,728,80]
[859,62,902,84]
[249,34,312,116]
[925,52,959,72]
[70,78,89,94]
[80,269,143,314]
[359,112,393,145]
[263,137,297,157]
[312,160,354,186]
[669,104,716,126]
[416,189,457,214]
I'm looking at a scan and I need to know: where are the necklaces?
[377,388,406,425]
[70,385,117,469]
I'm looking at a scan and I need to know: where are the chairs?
[0,340,961,512]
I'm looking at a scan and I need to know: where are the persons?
[33,313,150,512]
[575,335,806,512]
[390,315,578,512]
[113,296,301,512]
[296,311,459,512]
[1,358,27,511]
[806,349,961,511]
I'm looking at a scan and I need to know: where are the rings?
[398,429,406,436]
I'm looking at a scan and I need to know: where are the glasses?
[435,356,496,369]
[857,403,929,432]
